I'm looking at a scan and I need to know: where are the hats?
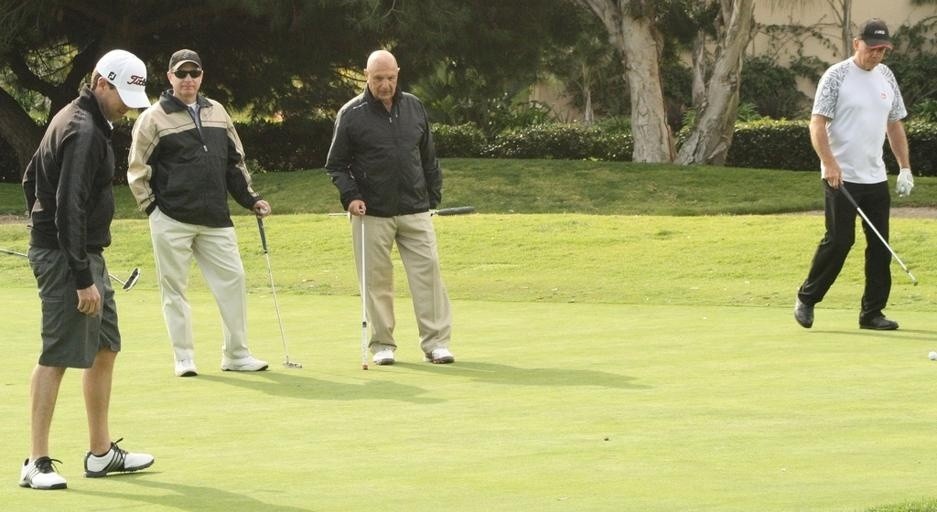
[168,48,202,73]
[856,16,894,51]
[94,47,152,109]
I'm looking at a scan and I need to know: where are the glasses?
[174,70,203,79]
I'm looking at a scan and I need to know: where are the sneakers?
[174,352,200,376]
[794,292,814,328]
[859,310,900,331]
[19,456,69,491]
[220,347,269,371]
[83,436,159,479]
[423,345,455,363]
[372,348,396,366]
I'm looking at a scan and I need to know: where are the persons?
[321,47,459,367]
[20,46,162,492]
[121,45,276,379]
[788,16,917,334]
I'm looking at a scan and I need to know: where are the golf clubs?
[256,209,305,373]
[2,245,140,290]
[360,207,371,372]
[838,182,918,288]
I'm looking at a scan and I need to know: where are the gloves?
[895,168,915,198]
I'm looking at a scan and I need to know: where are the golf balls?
[929,352,936,360]
[898,186,908,196]
